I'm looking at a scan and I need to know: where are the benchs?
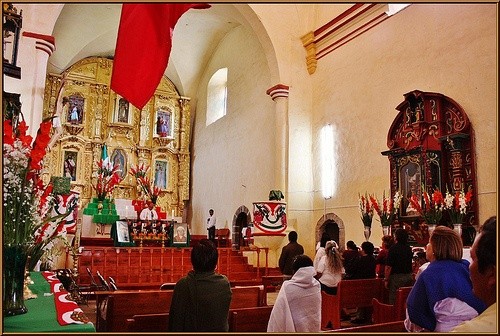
[80,277,413,332]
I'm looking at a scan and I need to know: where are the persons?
[415,252,430,281]
[168,238,232,332]
[313,228,412,296]
[64,154,75,177]
[113,153,123,176]
[70,106,80,122]
[119,101,128,118]
[160,116,167,132]
[267,255,321,332]
[173,226,187,242]
[406,217,497,333]
[207,209,216,240]
[118,222,128,241]
[278,231,304,282]
[156,166,165,188]
[140,203,158,220]
[415,107,422,121]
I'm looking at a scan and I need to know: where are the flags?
[111,4,212,110]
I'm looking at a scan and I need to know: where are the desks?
[112,219,178,246]
[94,214,119,236]
[3,270,96,333]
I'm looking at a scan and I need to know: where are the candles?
[172,210,175,219]
[125,208,128,218]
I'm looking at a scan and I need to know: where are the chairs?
[214,228,230,247]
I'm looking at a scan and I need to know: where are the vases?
[427,224,436,237]
[363,226,372,241]
[1,244,30,316]
[98,201,103,213]
[453,224,462,242]
[383,226,390,237]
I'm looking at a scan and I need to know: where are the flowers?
[128,163,163,199]
[359,182,473,227]
[89,158,121,201]
[4,117,78,259]
